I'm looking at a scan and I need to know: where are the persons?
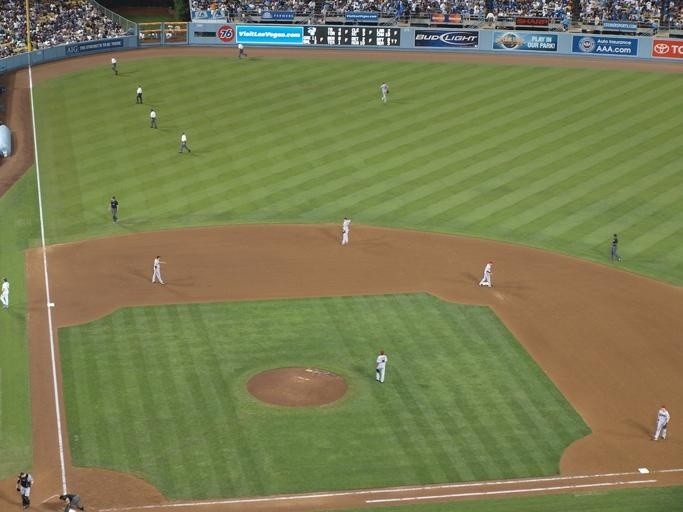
[338,218,352,244]
[41,491,84,512]
[139,24,182,42]
[149,107,158,129]
[178,131,191,154]
[375,349,388,383]
[134,83,144,103]
[651,405,672,441]
[107,194,121,225]
[0,1,130,61]
[192,1,683,37]
[477,262,493,288]
[609,233,624,266]
[150,256,167,285]
[0,278,9,306]
[236,42,249,59]
[376,82,394,103]
[110,57,117,76]
[14,472,36,509]
